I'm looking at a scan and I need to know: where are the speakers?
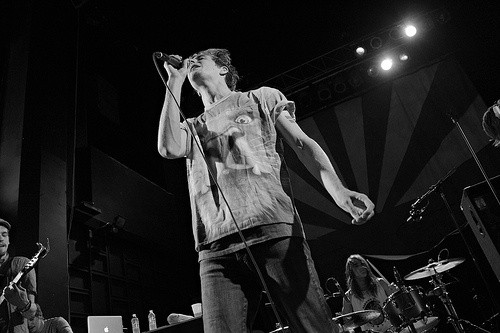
[460,175,500,282]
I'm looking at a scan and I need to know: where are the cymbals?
[404,257,465,280]
[333,310,381,331]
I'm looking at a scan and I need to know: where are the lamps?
[290,9,452,115]
[113,216,128,229]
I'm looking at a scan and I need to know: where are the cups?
[191,303,202,318]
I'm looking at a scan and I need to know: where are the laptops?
[87,316,124,333]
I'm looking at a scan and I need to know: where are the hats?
[0,219,11,229]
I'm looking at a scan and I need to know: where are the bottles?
[131,314,140,333]
[148,310,157,331]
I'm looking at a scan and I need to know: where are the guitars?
[0,242,48,333]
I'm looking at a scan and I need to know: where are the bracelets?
[17,300,32,314]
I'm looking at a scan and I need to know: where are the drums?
[382,285,440,333]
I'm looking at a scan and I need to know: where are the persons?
[27,304,75,333]
[0,219,37,333]
[342,253,396,316]
[155,48,376,333]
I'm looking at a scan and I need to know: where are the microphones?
[154,51,189,69]
[333,278,351,303]
[393,266,404,286]
